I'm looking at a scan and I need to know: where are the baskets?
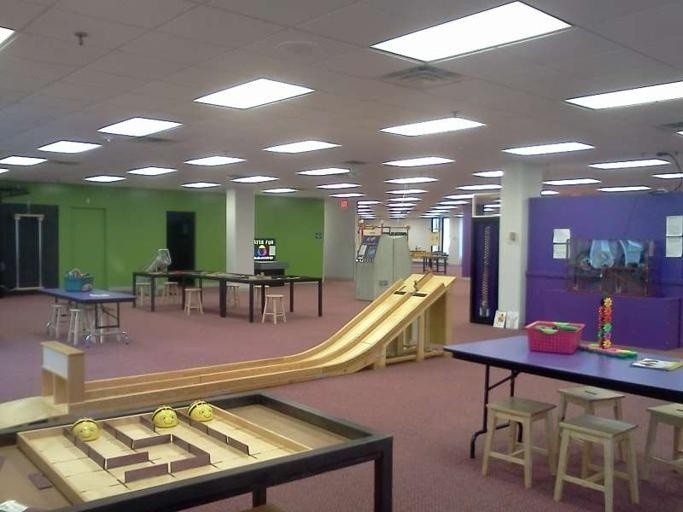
[525,321,586,353]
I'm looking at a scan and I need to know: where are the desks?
[443,334,682,459]
[422,255,446,275]
[0,391,394,512]
[39,287,137,348]
[131,270,322,323]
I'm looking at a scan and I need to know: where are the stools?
[49,301,120,346]
[226,285,286,325]
[480,384,682,511]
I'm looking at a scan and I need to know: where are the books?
[630,357,683,372]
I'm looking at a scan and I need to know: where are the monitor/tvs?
[366,245,376,258]
